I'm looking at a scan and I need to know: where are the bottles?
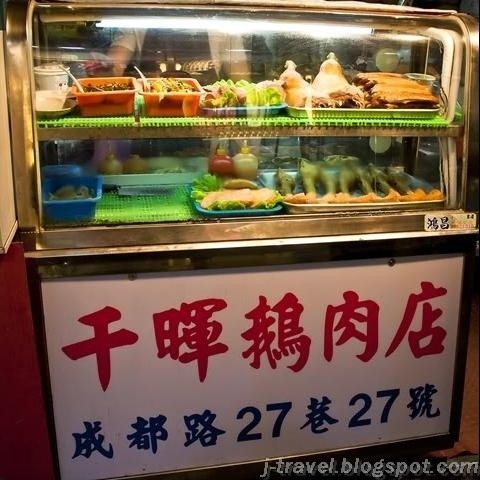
[209,148,257,181]
[99,154,148,174]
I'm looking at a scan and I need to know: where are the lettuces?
[191,175,283,210]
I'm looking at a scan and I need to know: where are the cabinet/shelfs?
[0,0,480,480]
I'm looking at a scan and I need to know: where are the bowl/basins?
[271,157,311,169]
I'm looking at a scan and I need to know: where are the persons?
[94,27,255,87]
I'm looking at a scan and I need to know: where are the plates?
[35,98,76,119]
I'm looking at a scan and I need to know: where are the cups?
[406,73,436,87]
[33,65,69,90]
